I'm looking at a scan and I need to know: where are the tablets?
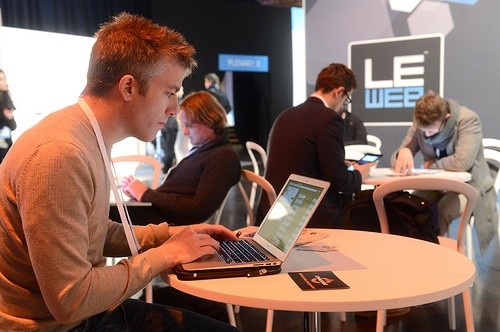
[402,169,444,174]
[347,153,383,172]
[109,201,152,206]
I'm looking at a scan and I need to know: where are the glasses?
[343,90,352,105]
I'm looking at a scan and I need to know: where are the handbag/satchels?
[339,188,441,245]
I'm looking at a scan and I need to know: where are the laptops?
[174,174,331,280]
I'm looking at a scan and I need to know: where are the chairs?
[232,170,278,313]
[445,137,500,260]
[104,156,160,304]
[368,176,479,332]
[245,142,268,224]
[367,135,382,168]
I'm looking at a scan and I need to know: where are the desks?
[159,228,476,332]
[360,168,475,288]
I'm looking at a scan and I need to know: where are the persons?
[204,73,232,115]
[155,117,179,175]
[390,89,498,257]
[0,12,242,332]
[254,63,411,318]
[0,69,17,166]
[109,90,241,226]
